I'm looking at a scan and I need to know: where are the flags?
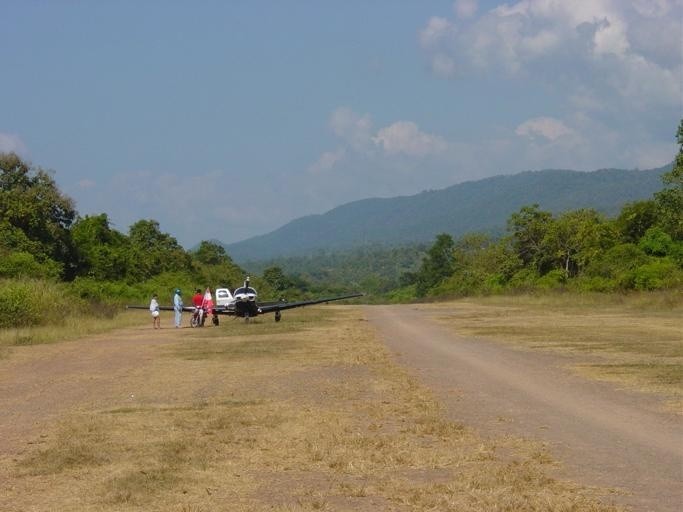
[201,285,214,314]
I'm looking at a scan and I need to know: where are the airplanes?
[124,277,363,324]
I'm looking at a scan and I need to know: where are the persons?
[192,288,204,328]
[150,293,162,330]
[174,289,183,328]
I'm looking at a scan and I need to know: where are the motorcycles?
[190,304,207,327]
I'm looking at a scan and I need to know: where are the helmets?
[175,289,181,295]
